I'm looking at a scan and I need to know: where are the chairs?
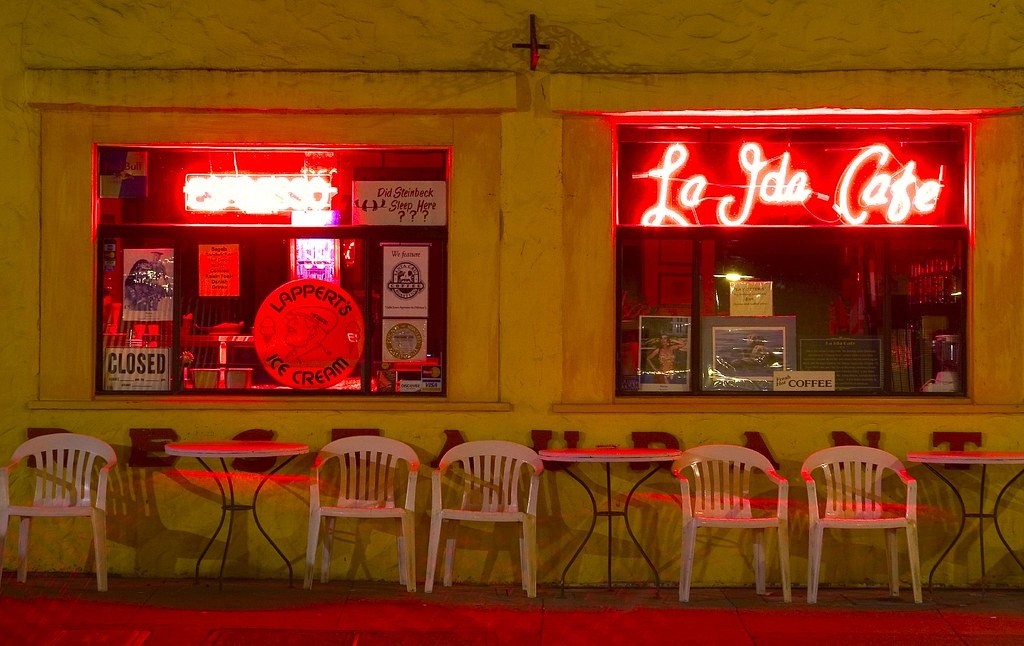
[671,443,792,605]
[423,440,545,599]
[801,445,924,605]
[0,432,119,593]
[301,434,420,594]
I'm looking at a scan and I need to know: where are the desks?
[537,447,683,600]
[905,451,1024,595]
[164,439,310,592]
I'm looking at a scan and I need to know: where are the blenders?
[931,333,963,393]
